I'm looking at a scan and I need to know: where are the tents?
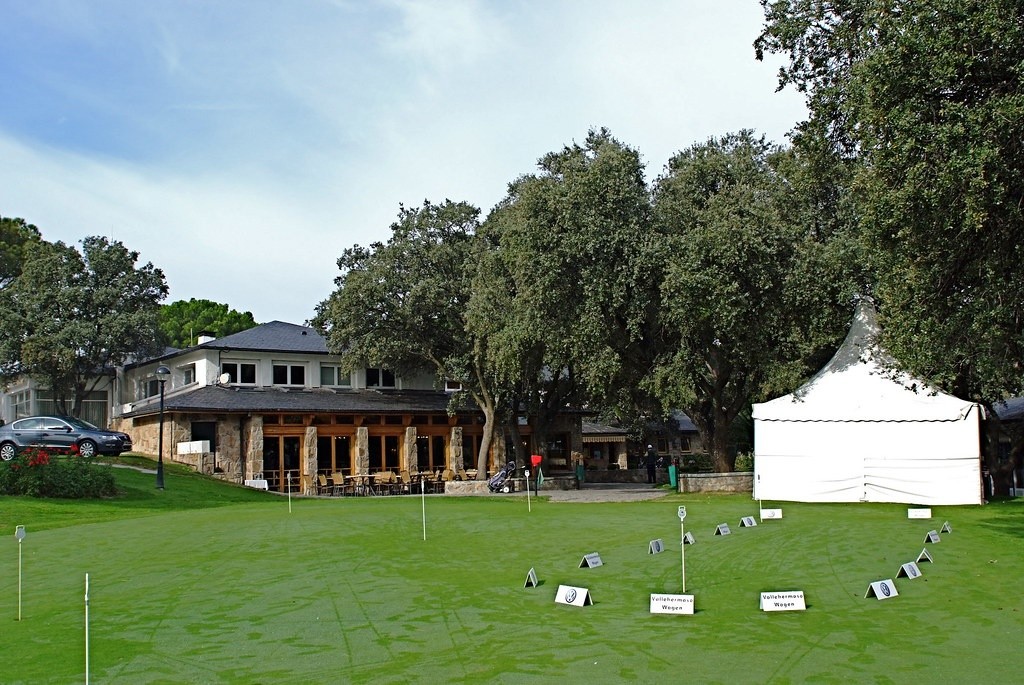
[752,298,984,505]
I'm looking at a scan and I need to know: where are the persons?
[644,445,656,483]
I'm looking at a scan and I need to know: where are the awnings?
[581,423,625,442]
[986,398,1024,421]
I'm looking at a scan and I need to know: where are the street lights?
[155,366,172,490]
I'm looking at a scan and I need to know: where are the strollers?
[488,461,516,493]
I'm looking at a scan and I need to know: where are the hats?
[647,445,652,449]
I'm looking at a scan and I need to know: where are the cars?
[628,452,644,469]
[657,455,680,467]
[0,415,133,462]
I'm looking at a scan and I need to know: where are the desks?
[245,479,269,490]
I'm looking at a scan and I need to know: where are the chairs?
[303,469,496,496]
